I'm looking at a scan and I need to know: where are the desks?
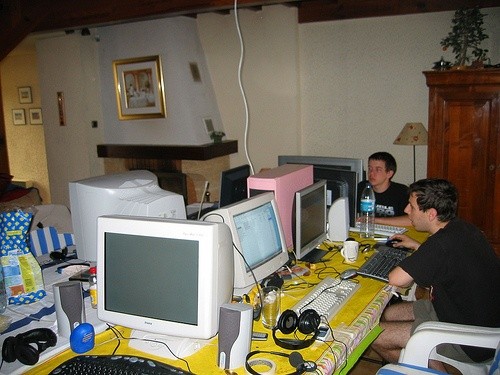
[0,219,430,375]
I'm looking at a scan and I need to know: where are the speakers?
[328,197,349,240]
[53,281,87,339]
[217,303,253,370]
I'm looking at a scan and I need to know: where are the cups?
[341,241,359,263]
[259,286,281,329]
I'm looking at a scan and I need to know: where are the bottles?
[360,183,375,239]
[0,258,7,314]
[89,267,97,309]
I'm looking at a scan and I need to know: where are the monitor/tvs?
[68,155,364,359]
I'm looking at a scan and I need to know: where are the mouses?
[340,269,358,280]
[385,238,410,251]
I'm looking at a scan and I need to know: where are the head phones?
[271,309,321,350]
[245,351,318,375]
[2,328,58,366]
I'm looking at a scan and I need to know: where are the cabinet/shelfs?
[421,69,500,253]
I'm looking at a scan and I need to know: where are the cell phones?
[251,332,268,340]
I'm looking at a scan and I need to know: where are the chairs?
[374,321,500,375]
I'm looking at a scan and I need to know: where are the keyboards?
[349,222,407,236]
[357,245,407,283]
[290,277,361,326]
[48,354,196,375]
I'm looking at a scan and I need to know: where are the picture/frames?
[112,53,168,120]
[29,107,42,125]
[18,86,33,104]
[203,117,215,135]
[11,108,27,125]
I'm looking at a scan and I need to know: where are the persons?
[370,178,500,374]
[356,152,414,228]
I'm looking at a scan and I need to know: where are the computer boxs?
[247,163,313,248]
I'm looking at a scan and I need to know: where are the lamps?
[393,122,428,182]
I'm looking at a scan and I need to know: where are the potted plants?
[440,9,491,71]
